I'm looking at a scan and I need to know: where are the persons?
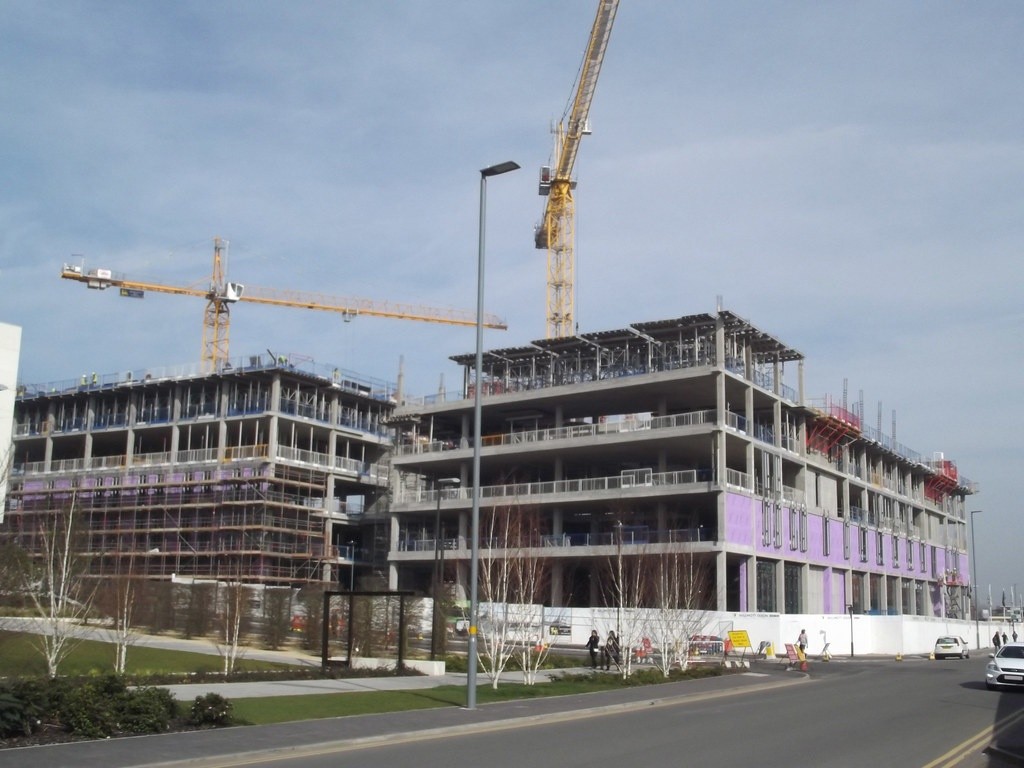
[604,631,620,671]
[796,629,808,659]
[584,630,599,670]
[1002,631,1007,645]
[993,631,1001,652]
[1012,630,1018,642]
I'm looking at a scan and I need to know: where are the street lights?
[465,159,521,710]
[348,540,356,591]
[429,478,460,660]
[971,509,982,650]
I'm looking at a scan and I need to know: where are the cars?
[934,635,969,660]
[985,642,1024,690]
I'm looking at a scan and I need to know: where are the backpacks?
[993,637,997,644]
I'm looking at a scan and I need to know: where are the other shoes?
[617,668,619,671]
[606,666,610,670]
[805,653,807,658]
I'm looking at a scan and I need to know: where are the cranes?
[533,1,621,340]
[61,238,508,377]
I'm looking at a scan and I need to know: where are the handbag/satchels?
[593,648,599,652]
